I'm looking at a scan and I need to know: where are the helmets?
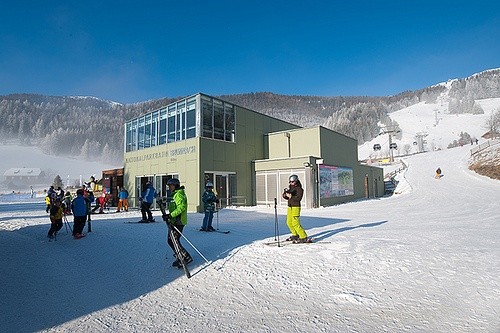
[77,190,83,195]
[167,178,180,189]
[146,180,152,186]
[289,175,298,182]
[205,182,214,188]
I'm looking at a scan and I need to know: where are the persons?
[85,176,96,190]
[116,187,129,212]
[282,175,310,242]
[71,189,95,239]
[45,193,51,212]
[202,183,220,231]
[92,195,113,213]
[55,187,64,198]
[47,199,65,239]
[435,166,442,177]
[82,185,95,234]
[162,178,192,267]
[63,191,72,215]
[47,186,56,199]
[139,181,158,223]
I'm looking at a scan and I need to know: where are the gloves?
[158,196,167,209]
[61,202,65,208]
[207,199,211,203]
[215,198,220,203]
[45,205,50,213]
[162,213,171,221]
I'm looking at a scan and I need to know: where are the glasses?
[289,180,295,182]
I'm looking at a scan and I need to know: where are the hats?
[54,197,62,202]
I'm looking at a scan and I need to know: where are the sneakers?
[77,232,84,236]
[178,255,193,268]
[202,226,211,231]
[286,234,299,240]
[47,233,54,238]
[74,234,79,239]
[172,258,180,266]
[146,218,155,222]
[139,219,148,222]
[294,237,308,243]
[208,226,215,231]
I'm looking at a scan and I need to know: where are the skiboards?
[263,241,331,247]
[86,192,91,232]
[191,228,232,234]
[123,222,162,224]
[156,198,191,278]
